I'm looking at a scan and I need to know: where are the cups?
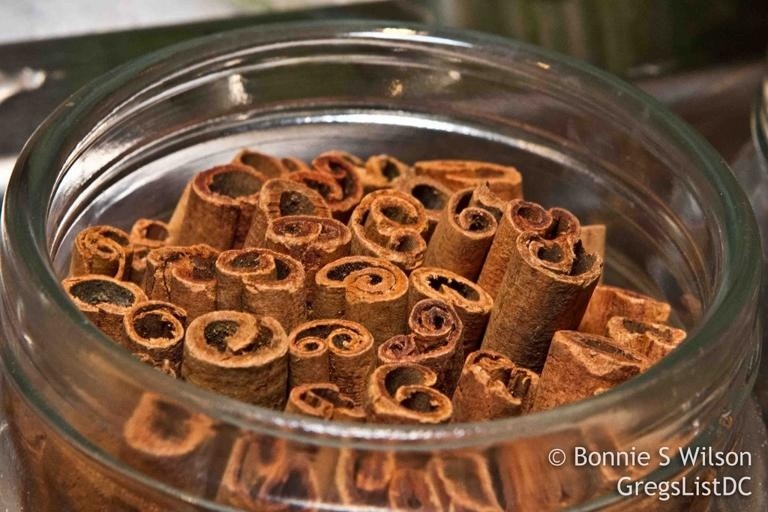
[1,19,765,509]
